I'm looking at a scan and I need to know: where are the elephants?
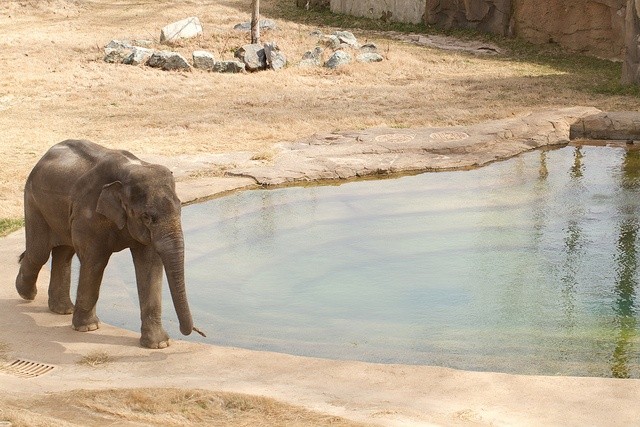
[16,139,192,349]
